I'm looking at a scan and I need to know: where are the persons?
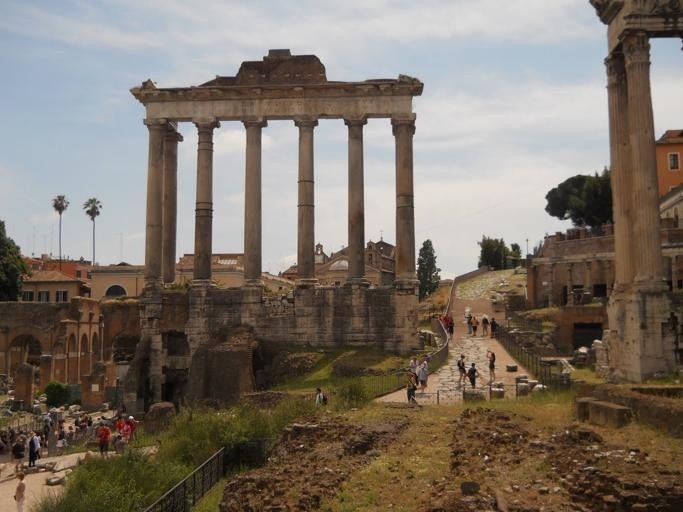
[404,356,429,404]
[487,350,495,385]
[0,397,141,473]
[464,312,499,338]
[467,363,479,389]
[315,387,323,406]
[458,354,466,387]
[16,472,26,512]
[438,313,454,336]
